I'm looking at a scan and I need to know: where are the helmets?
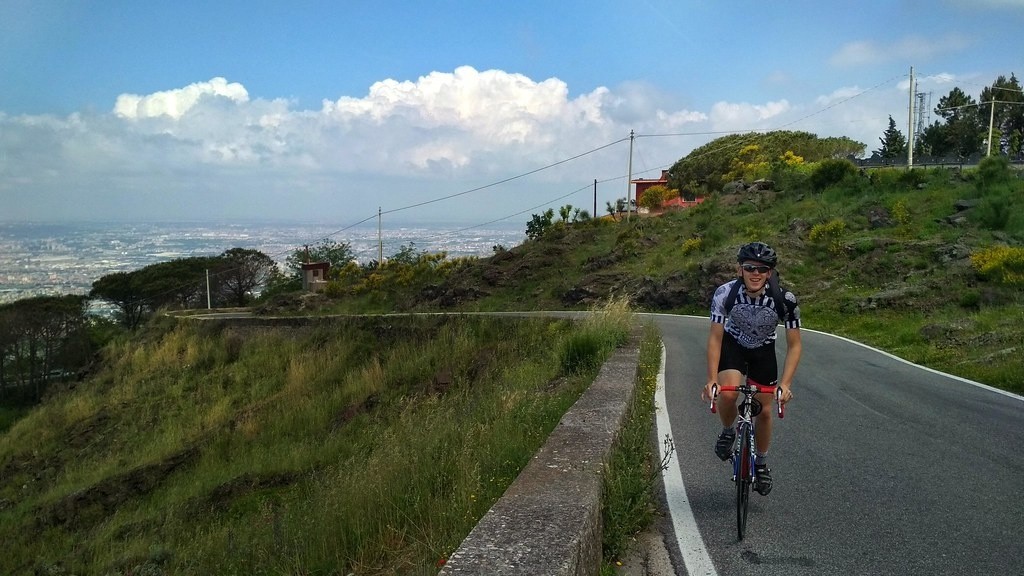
[736,241,778,268]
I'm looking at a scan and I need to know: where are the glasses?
[741,263,770,274]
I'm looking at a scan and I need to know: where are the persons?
[701,242,801,496]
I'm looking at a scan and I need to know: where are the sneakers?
[755,462,772,495]
[713,431,736,459]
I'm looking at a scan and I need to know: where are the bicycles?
[710,383,785,540]
